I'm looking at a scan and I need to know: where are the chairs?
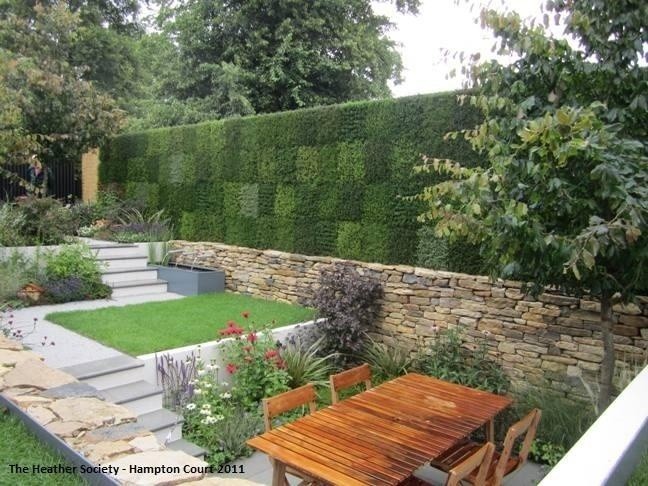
[430,408,541,485]
[397,442,495,486]
[263,384,328,485]
[330,362,371,404]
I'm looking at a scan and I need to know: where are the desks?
[244,372,514,486]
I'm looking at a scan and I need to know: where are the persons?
[27,155,53,199]
